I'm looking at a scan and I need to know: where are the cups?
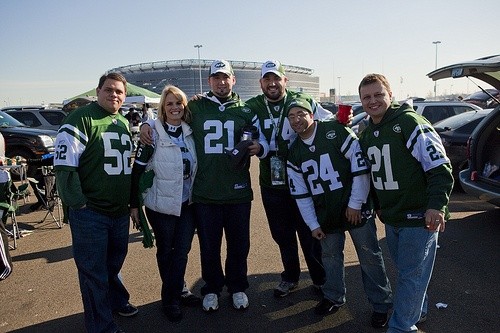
[338,105,352,124]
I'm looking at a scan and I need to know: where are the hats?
[223,140,253,170]
[209,59,233,77]
[261,59,286,79]
[286,98,313,117]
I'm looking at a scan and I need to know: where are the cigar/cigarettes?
[435,220,447,222]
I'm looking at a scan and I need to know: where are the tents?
[62,81,161,110]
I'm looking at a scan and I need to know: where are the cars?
[318,54,500,207]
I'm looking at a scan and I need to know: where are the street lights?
[194,45,203,97]
[433,41,443,101]
[338,76,342,104]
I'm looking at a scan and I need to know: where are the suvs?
[0,106,71,178]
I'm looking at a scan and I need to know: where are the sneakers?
[202,293,219,313]
[232,291,250,312]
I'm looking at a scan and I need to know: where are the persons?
[54,60,269,333]
[0,132,12,280]
[358,76,455,333]
[283,98,395,329]
[191,60,354,298]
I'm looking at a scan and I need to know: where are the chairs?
[0,170,20,249]
[25,165,64,228]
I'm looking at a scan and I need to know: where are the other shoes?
[371,312,389,328]
[162,287,201,323]
[118,301,138,317]
[274,280,299,297]
[313,298,345,315]
[314,283,322,289]
